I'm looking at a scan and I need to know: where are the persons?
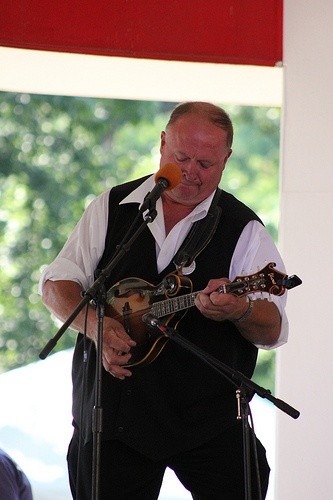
[41,103,289,500]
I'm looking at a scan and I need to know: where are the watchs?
[236,294,253,324]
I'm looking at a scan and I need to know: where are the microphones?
[151,318,170,338]
[139,163,182,213]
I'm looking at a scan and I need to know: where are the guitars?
[93,262,303,374]
